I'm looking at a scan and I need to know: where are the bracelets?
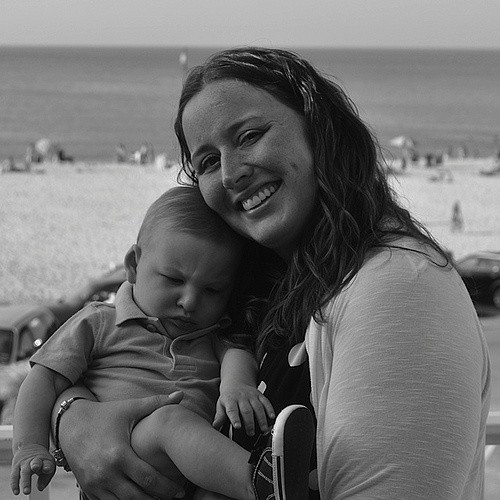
[52,395,92,473]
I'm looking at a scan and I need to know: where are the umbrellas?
[388,135,421,150]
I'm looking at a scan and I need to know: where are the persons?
[394,141,477,183]
[0,137,75,175]
[448,197,464,232]
[45,49,492,500]
[115,139,172,170]
[8,185,321,500]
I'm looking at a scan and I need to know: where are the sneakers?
[248,404,316,500]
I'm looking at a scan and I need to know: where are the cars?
[76,266,127,312]
[455,250,500,311]
[0,304,61,465]
